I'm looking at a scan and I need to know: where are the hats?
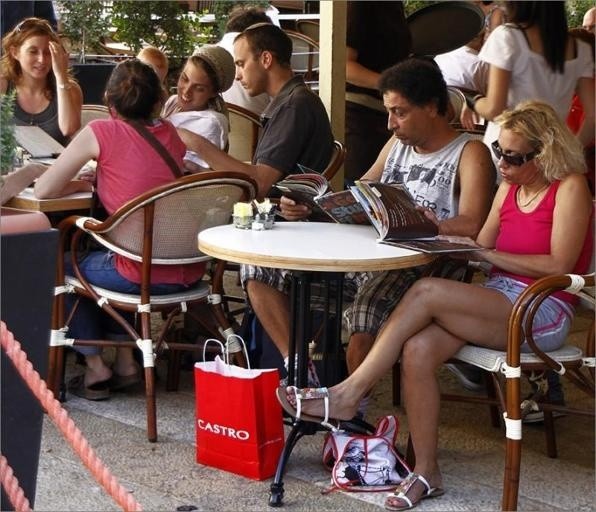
[192,45,235,92]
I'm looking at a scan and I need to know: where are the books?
[271,163,372,226]
[347,179,487,255]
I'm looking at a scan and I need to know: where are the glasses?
[491,141,539,166]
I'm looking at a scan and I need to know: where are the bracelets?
[57,82,76,90]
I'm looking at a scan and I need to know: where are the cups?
[258,202,277,229]
[231,214,253,229]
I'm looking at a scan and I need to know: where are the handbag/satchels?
[196,334,285,480]
[330,434,404,486]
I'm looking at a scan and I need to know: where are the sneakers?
[520,392,568,423]
[443,358,486,391]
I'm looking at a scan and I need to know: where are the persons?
[1,18,84,149]
[164,23,331,370]
[160,43,236,175]
[35,56,200,398]
[344,0,416,184]
[237,57,497,406]
[2,0,61,35]
[135,48,171,103]
[216,9,272,116]
[429,2,595,196]
[278,100,594,506]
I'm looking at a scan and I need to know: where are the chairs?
[41,170,261,442]
[280,29,319,98]
[67,103,113,141]
[406,200,596,512]
[219,103,269,162]
[295,19,318,82]
[210,139,349,389]
[308,183,499,406]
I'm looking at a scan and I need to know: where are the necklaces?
[518,183,550,207]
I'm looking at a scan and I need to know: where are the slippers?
[108,362,142,392]
[68,373,110,400]
[276,386,346,433]
[385,471,444,511]
